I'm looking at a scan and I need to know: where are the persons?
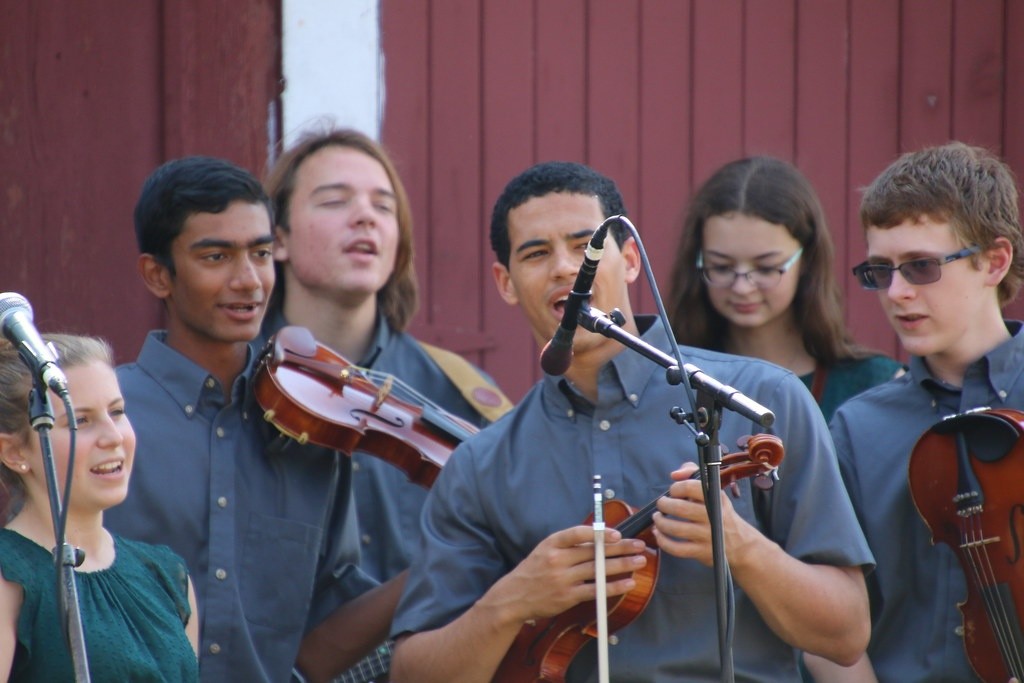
[797,142,1024,683]
[386,158,876,683]
[668,156,911,432]
[259,126,519,431]
[109,157,413,683]
[0,333,201,683]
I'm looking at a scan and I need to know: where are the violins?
[254,324,479,488]
[488,431,785,683]
[906,407,1024,683]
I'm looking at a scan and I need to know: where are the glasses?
[853,245,980,291]
[697,247,803,290]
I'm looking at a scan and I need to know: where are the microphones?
[540,224,609,376]
[0,292,68,397]
